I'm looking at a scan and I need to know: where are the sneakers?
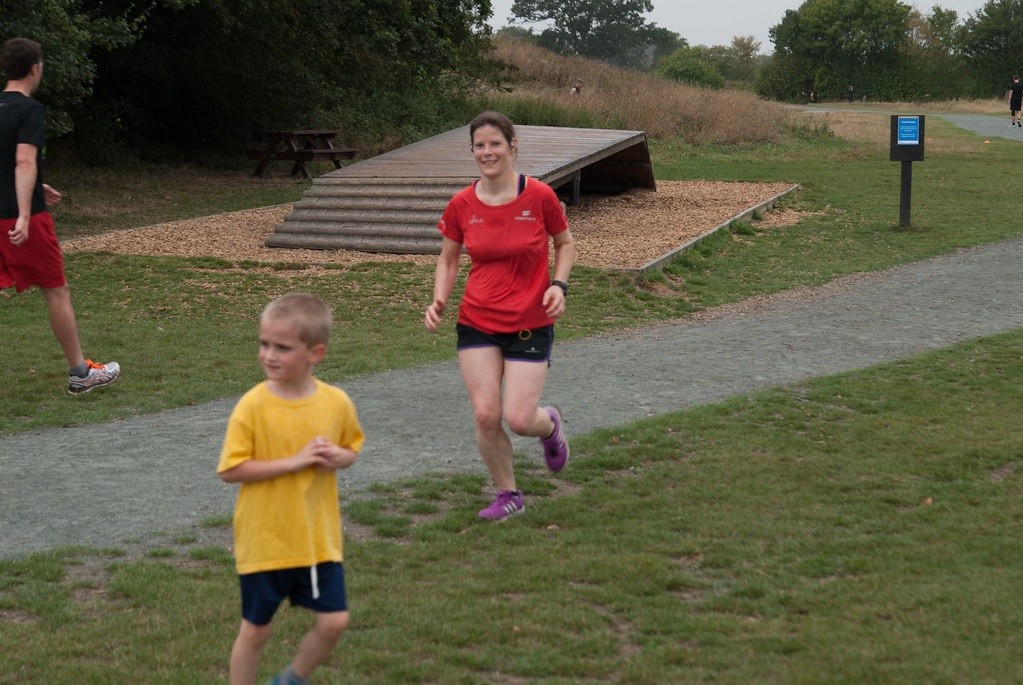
[541,402,569,473]
[479,487,526,522]
[69,360,121,395]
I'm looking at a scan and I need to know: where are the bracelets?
[552,280,568,297]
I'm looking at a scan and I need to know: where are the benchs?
[248,129,361,180]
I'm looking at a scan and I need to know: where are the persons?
[217,290,365,685]
[0,37,122,396]
[424,111,576,525]
[1008,75,1023,129]
